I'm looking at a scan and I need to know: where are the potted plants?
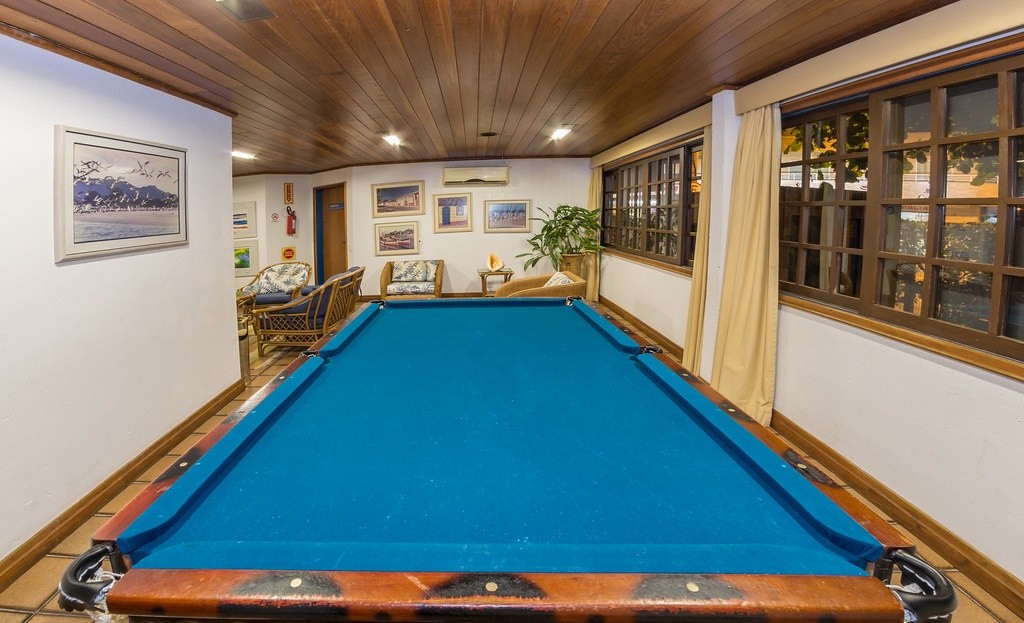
[514,203,607,280]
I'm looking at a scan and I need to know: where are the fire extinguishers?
[286,205,296,234]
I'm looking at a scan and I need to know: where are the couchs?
[379,259,444,300]
[494,270,587,299]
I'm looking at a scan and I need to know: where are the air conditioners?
[442,166,510,186]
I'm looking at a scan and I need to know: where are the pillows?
[543,271,574,286]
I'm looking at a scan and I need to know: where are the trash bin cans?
[237,316,251,383]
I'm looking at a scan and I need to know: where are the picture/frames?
[53,125,190,264]
[483,200,531,232]
[432,193,472,234]
[373,221,421,255]
[371,180,426,219]
[232,200,258,239]
[234,240,260,275]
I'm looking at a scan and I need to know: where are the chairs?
[236,262,365,356]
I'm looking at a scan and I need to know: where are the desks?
[57,295,959,623]
[236,291,257,336]
[478,268,515,298]
